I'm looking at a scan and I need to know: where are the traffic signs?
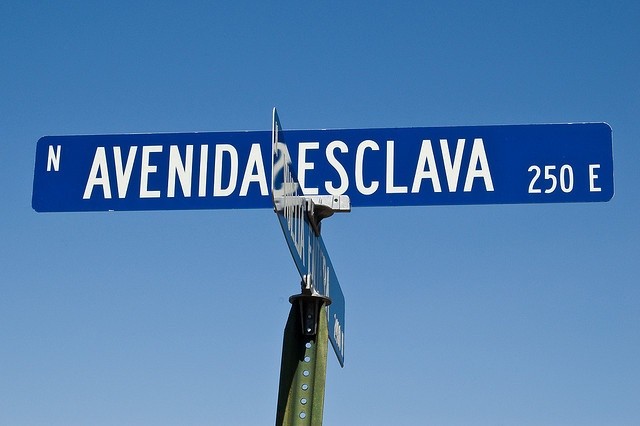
[269,106,347,368]
[32,120,614,212]
